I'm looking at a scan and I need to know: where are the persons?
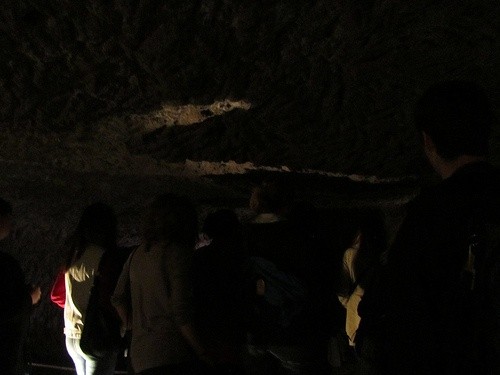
[48,199,130,375]
[194,75,499,374]
[109,191,222,375]
[0,198,42,375]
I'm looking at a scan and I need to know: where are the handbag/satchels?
[80,248,128,359]
